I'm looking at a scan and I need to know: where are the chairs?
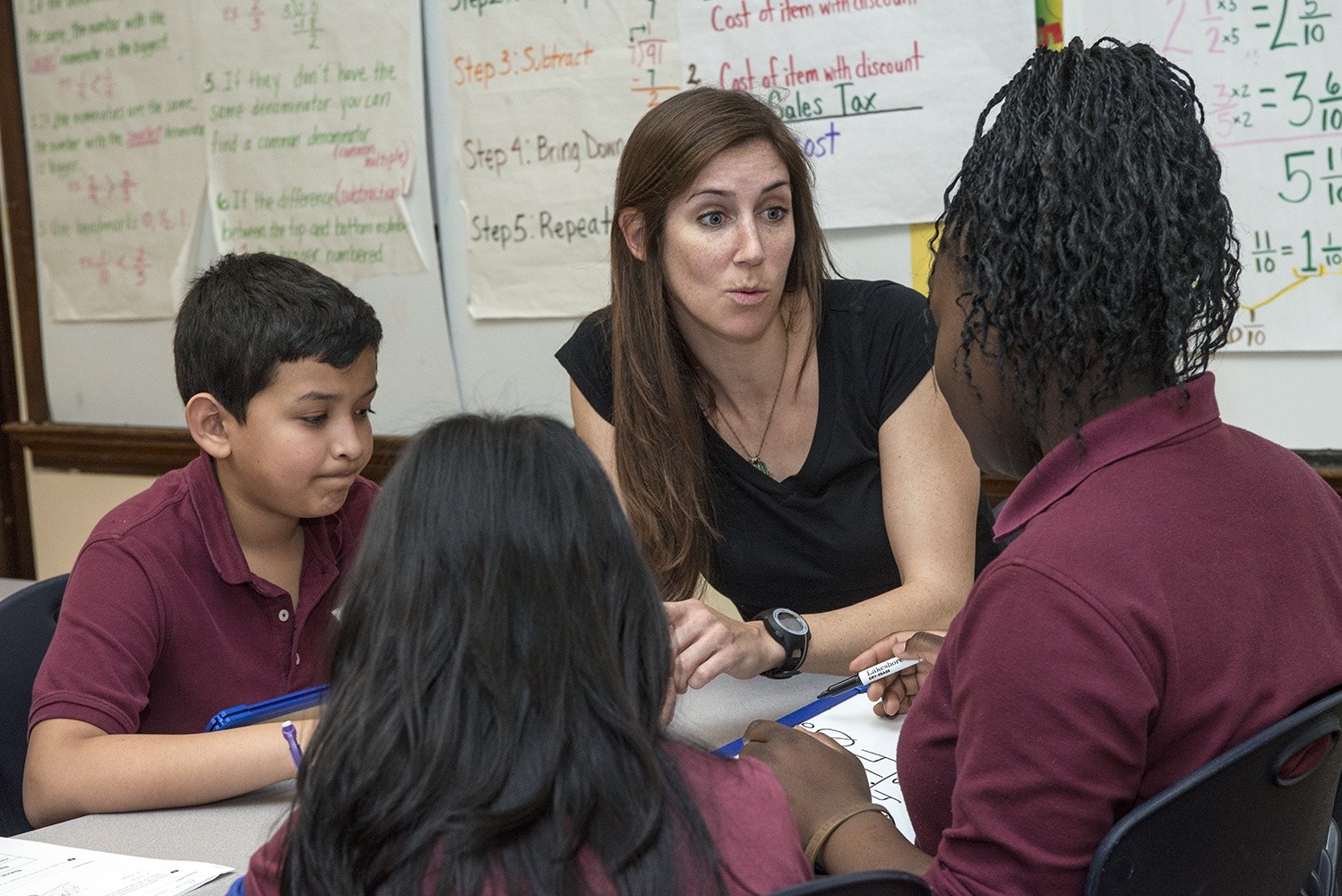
[1085,693,1342,896]
[0,573,74,837]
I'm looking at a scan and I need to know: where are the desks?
[0,673,915,896]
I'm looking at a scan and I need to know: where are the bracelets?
[802,803,895,880]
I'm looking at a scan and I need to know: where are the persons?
[24,249,385,827]
[554,87,997,690]
[226,410,810,896]
[736,38,1342,896]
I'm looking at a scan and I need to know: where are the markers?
[817,656,922,699]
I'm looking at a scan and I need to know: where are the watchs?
[749,606,812,680]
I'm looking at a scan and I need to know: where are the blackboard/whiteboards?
[0,2,1340,497]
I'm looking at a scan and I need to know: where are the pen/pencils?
[282,720,303,770]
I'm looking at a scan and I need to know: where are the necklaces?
[709,311,789,476]
